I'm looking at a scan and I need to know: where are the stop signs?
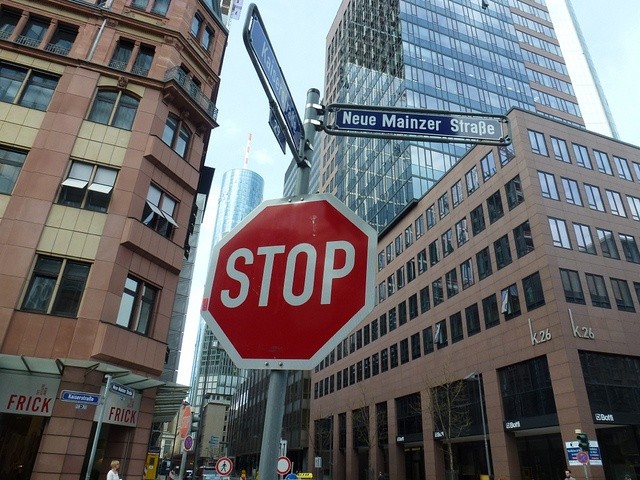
[200,194,379,371]
[179,405,191,437]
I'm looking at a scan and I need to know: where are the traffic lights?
[576,434,588,451]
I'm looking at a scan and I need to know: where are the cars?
[161,461,172,475]
[184,470,193,479]
[193,466,221,480]
[174,466,180,474]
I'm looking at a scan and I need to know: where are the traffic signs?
[59,389,104,406]
[109,381,135,398]
[242,2,306,164]
[324,103,513,146]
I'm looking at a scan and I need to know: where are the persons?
[564,470,575,479]
[107,460,122,480]
